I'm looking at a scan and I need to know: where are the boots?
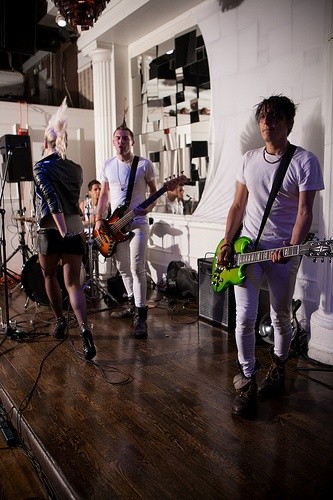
[109,297,139,320]
[258,345,291,397]
[233,360,258,415]
[133,305,149,340]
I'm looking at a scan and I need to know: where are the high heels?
[80,324,97,359]
[52,315,68,339]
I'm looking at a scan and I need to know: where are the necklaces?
[116,155,133,191]
[263,147,284,164]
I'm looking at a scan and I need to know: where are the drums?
[20,254,86,305]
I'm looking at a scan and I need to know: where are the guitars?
[211,236,333,293]
[92,171,188,258]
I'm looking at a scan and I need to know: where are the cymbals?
[13,216,36,223]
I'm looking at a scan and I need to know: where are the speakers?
[0,134,34,183]
[197,256,237,333]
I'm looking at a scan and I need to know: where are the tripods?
[0,182,50,348]
[76,196,122,307]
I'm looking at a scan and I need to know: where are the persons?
[34,98,96,359]
[95,98,158,329]
[167,183,184,214]
[80,179,113,280]
[217,96,325,415]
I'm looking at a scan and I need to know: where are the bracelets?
[220,243,231,250]
[97,219,107,221]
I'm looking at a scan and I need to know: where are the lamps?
[55,11,67,28]
